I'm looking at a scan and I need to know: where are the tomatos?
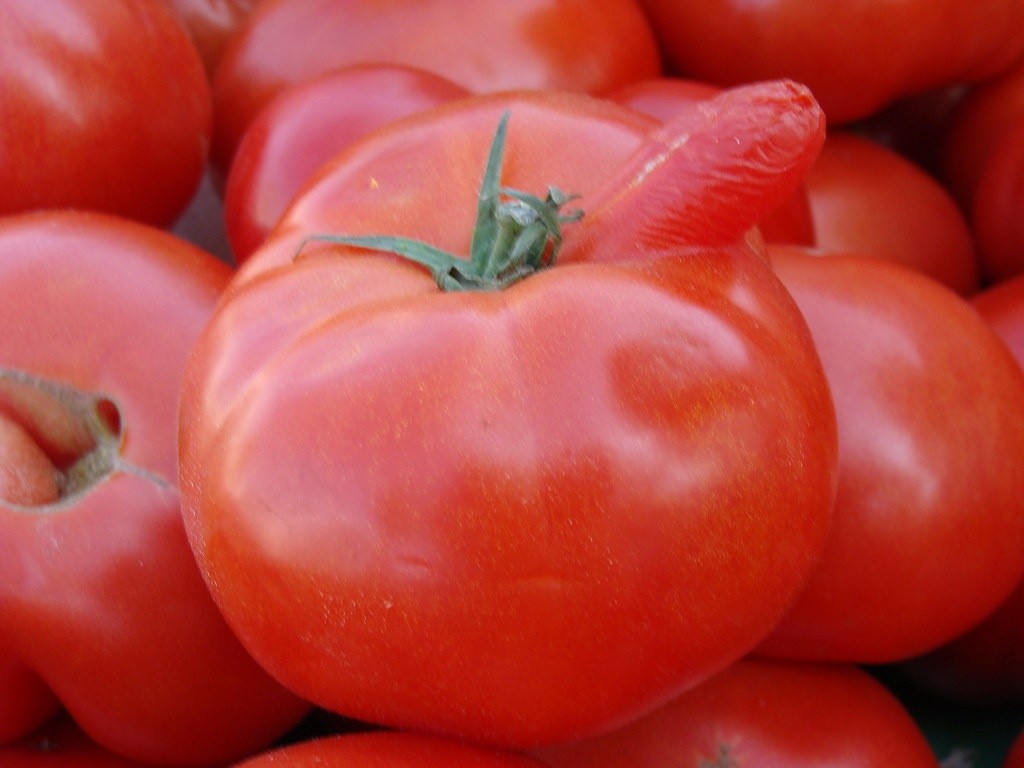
[0,0,1024,768]
